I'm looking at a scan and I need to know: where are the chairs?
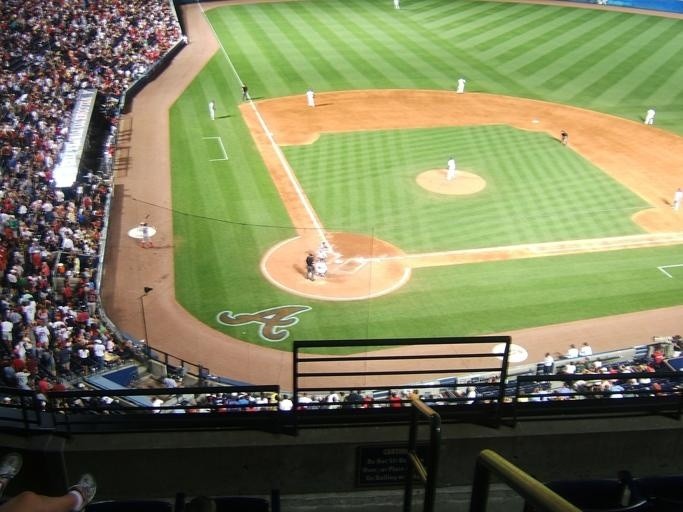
[187,487,282,511]
[86,498,187,511]
[525,473,629,511]
[628,477,682,512]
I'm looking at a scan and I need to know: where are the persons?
[670,188,683,211]
[393,0,401,9]
[315,242,329,260]
[306,88,316,107]
[643,107,657,125]
[445,156,457,182]
[305,253,316,281]
[558,129,571,147]
[456,78,467,95]
[315,257,329,278]
[0,449,98,512]
[1,0,190,413]
[192,332,683,414]
[241,82,249,102]
[208,98,217,121]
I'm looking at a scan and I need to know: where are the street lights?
[139,286,152,359]
